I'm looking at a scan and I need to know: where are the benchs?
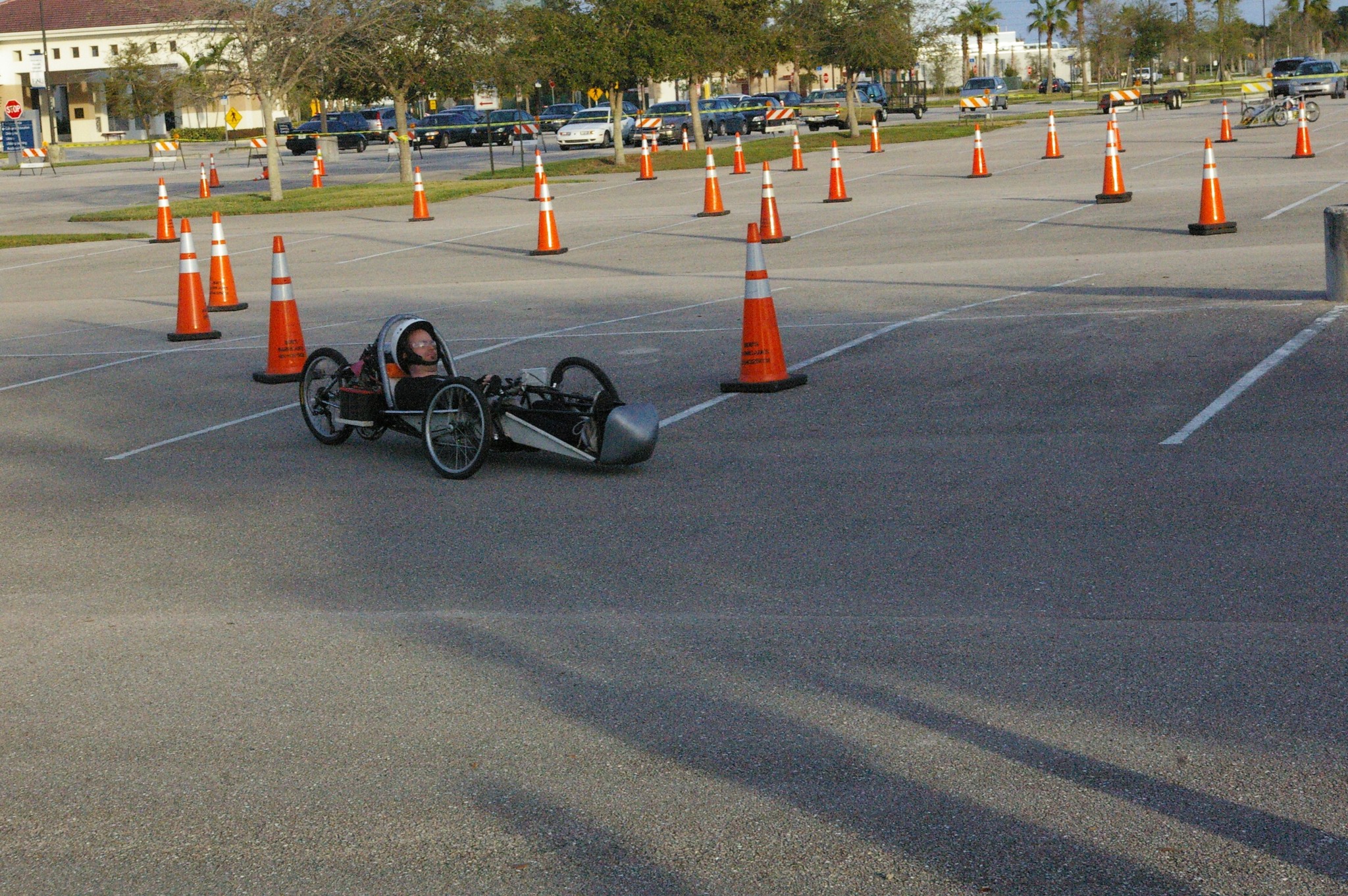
[102,133,126,140]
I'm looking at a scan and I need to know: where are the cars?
[540,88,823,152]
[1269,57,1346,100]
[285,120,368,156]
[1038,78,1071,94]
[412,105,541,148]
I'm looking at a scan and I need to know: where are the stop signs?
[5,100,22,119]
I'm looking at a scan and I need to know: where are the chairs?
[1306,68,1313,74]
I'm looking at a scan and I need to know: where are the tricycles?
[298,314,659,482]
[1240,91,1320,129]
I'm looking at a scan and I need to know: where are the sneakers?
[572,391,614,454]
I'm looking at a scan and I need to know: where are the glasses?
[409,341,437,348]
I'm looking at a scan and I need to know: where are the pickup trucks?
[799,90,889,125]
[1130,67,1159,86]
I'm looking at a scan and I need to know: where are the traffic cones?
[1188,138,1237,237]
[1041,109,1065,160]
[678,126,694,153]
[526,148,569,257]
[698,114,886,245]
[408,164,434,220]
[1214,100,1238,143]
[1095,107,1132,204]
[636,128,661,181]
[147,153,308,383]
[721,221,809,393]
[1291,101,1316,158]
[967,124,992,178]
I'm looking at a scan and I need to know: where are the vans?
[957,76,1008,112]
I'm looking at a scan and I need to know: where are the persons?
[391,318,614,454]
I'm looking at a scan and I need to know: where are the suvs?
[310,106,421,146]
[836,81,889,121]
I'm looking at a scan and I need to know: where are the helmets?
[390,318,437,376]
